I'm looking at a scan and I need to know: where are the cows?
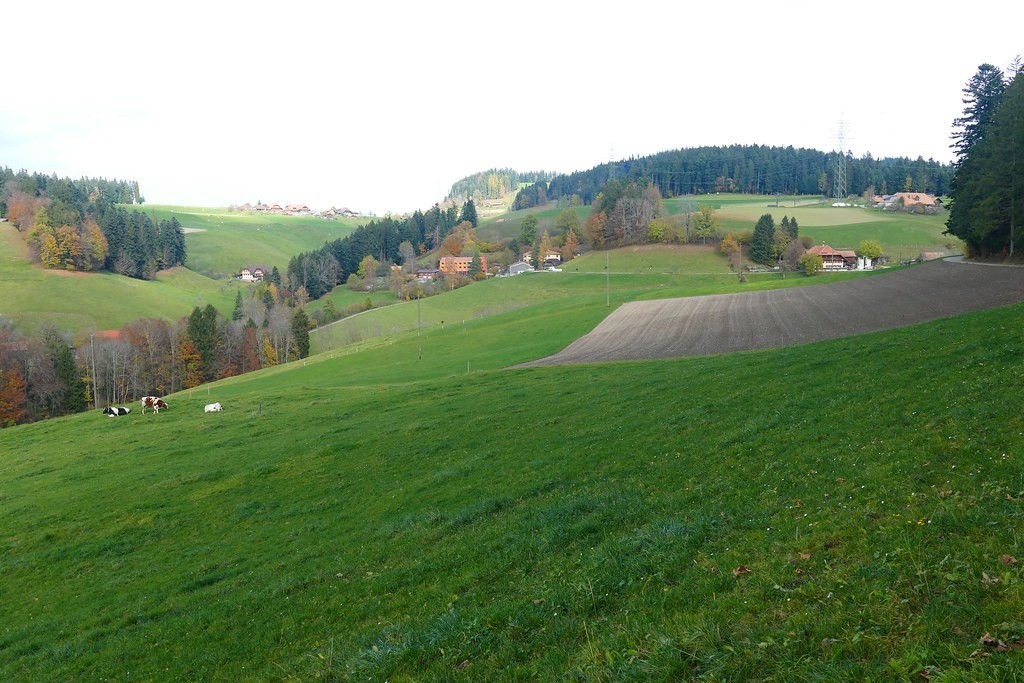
[141,396,169,414]
[102,406,131,417]
[203,402,224,413]
